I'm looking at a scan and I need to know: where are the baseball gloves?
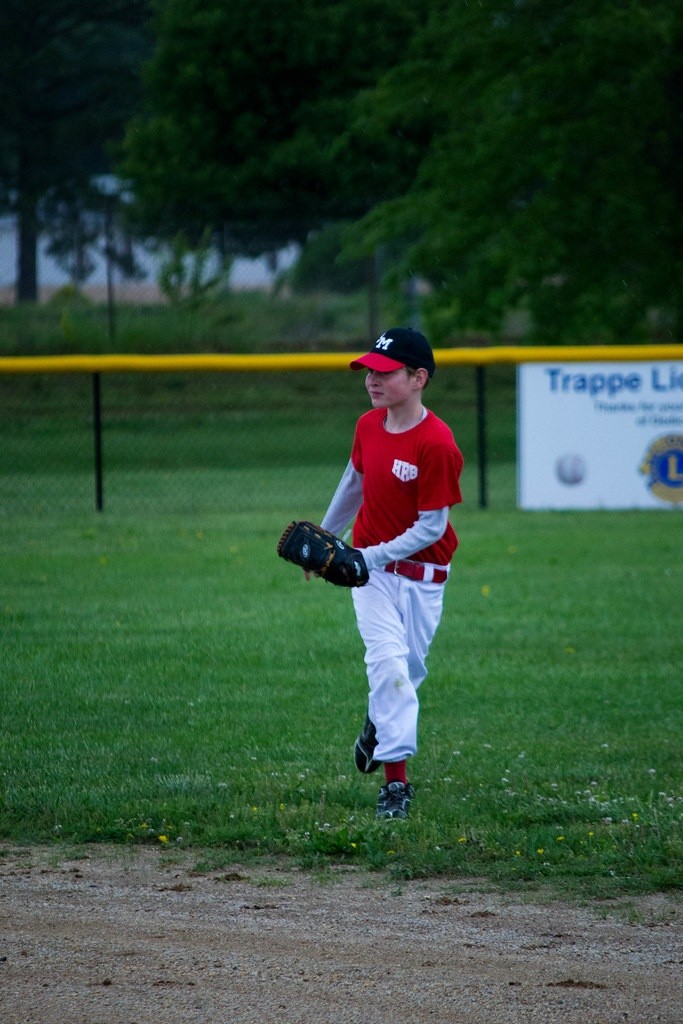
[276,519,369,589]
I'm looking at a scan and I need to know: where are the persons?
[304,327,465,822]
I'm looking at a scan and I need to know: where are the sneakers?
[352,713,386,774]
[376,779,416,823]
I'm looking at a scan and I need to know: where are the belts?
[384,560,448,584]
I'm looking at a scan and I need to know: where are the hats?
[349,327,436,379]
